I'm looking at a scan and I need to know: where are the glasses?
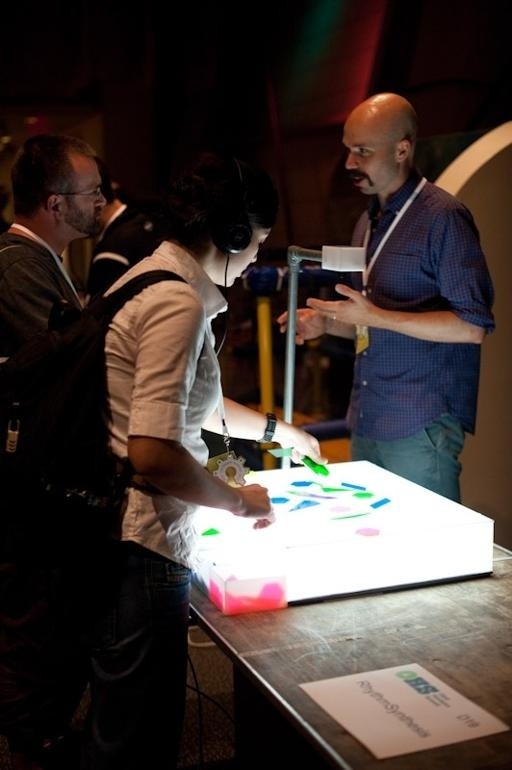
[55,186,102,201]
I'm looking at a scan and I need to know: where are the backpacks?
[0,268,189,568]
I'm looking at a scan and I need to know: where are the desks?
[185,539,512,770]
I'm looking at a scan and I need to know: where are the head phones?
[220,155,253,250]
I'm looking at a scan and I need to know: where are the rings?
[332,312,337,321]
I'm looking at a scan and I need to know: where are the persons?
[276,91,498,505]
